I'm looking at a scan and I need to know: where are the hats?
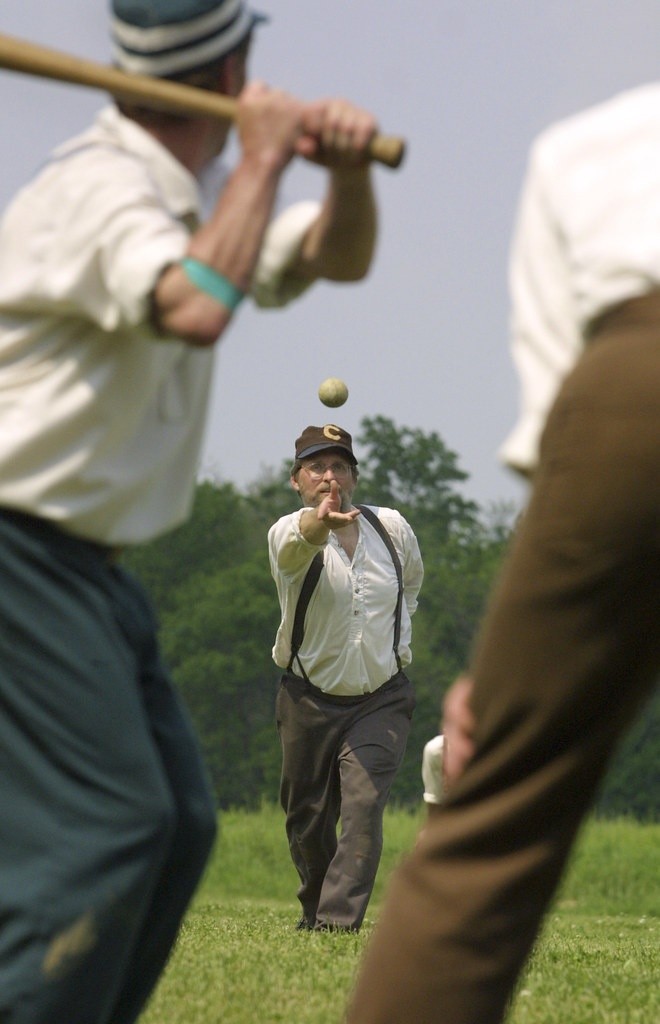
[107,0,266,79]
[295,424,358,465]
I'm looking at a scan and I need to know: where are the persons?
[267,424,424,936]
[353,81,660,1024]
[0,1,376,1024]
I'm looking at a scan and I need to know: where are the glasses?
[301,463,351,480]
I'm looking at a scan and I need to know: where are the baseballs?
[318,376,350,409]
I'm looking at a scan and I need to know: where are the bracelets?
[181,252,242,313]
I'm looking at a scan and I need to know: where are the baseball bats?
[0,27,411,169]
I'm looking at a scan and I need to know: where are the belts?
[0,508,122,564]
